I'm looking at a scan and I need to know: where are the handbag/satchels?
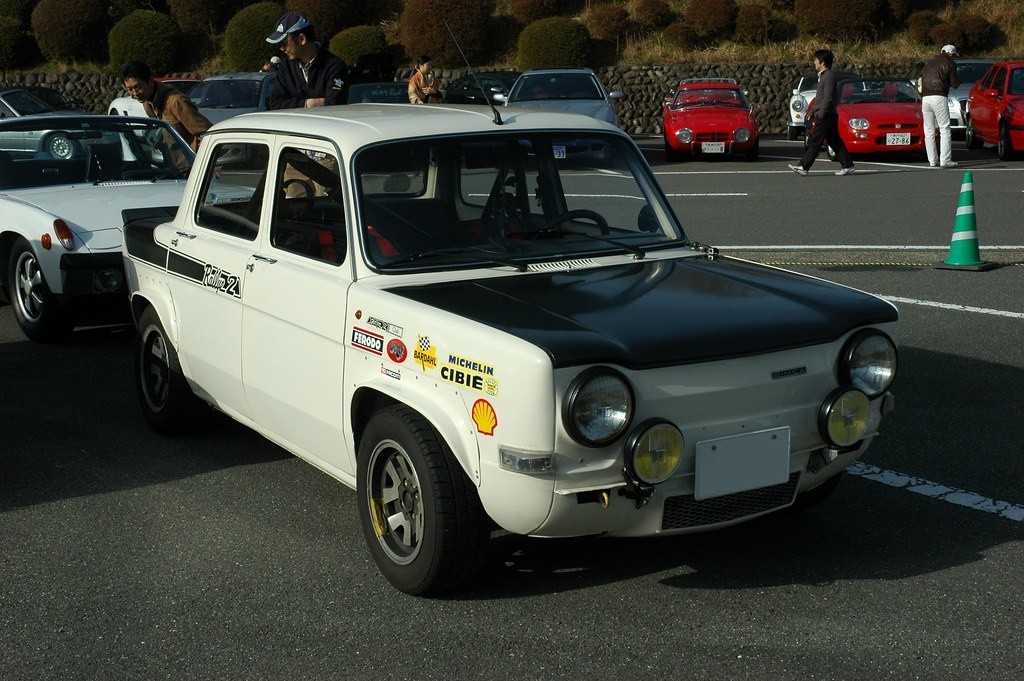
[917,76,923,94]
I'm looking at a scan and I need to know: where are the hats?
[266,12,309,44]
[940,44,960,57]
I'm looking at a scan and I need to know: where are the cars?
[493,67,624,129]
[347,82,412,105]
[107,77,201,118]
[803,77,940,162]
[0,86,87,114]
[445,71,523,106]
[0,114,293,341]
[910,58,1000,139]
[185,71,298,126]
[786,76,819,141]
[964,59,1024,161]
[119,100,902,598]
[662,77,760,162]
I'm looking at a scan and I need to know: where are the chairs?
[84,143,125,181]
[881,84,898,97]
[840,84,854,102]
[678,93,741,106]
[0,151,31,189]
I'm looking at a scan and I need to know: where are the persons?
[921,44,963,168]
[122,60,222,180]
[787,50,855,176]
[256,60,271,93]
[268,56,281,73]
[408,55,440,187]
[264,13,348,198]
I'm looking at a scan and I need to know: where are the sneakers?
[835,166,855,176]
[787,164,808,177]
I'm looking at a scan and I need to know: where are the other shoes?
[932,161,941,166]
[942,162,958,167]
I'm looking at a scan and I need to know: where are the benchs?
[323,219,526,261]
[217,197,460,251]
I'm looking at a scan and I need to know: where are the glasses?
[281,36,296,47]
[126,81,146,93]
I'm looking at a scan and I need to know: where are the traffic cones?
[943,170,985,267]
